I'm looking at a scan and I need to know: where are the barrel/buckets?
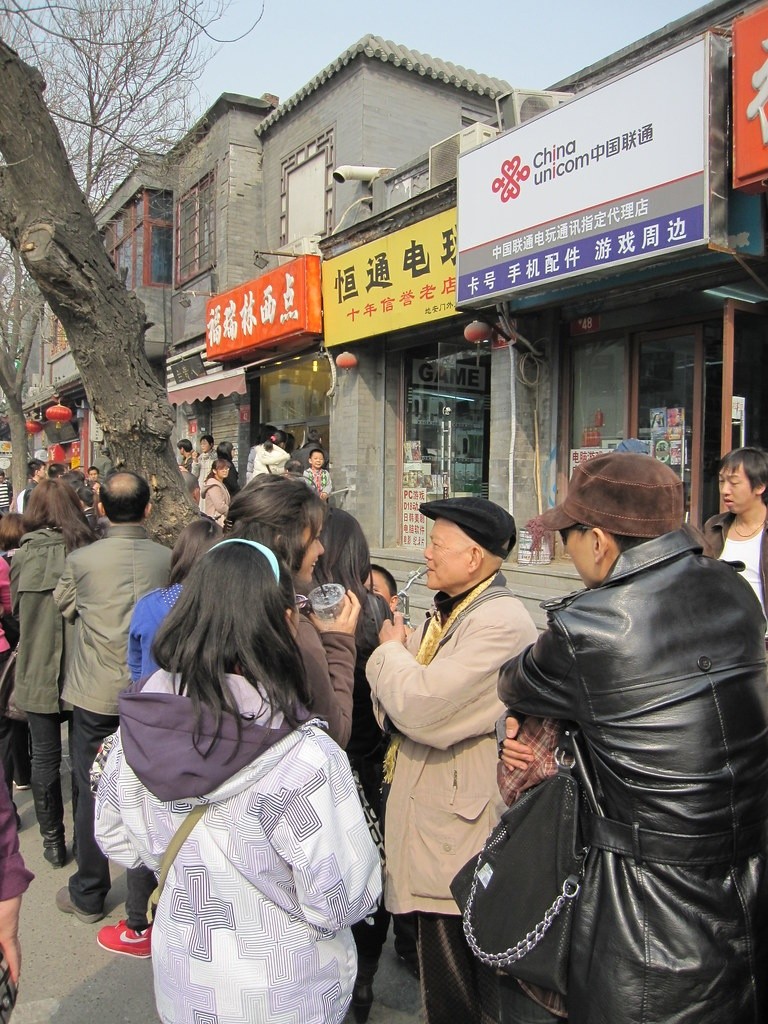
[518,528,552,565]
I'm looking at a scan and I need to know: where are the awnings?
[168,349,317,405]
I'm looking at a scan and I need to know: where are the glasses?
[215,467,230,472]
[291,594,309,608]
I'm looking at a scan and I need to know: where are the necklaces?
[735,516,765,538]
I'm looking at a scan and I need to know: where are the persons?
[170,424,332,525]
[1,459,224,961]
[92,474,422,1024]
[704,446,767,642]
[362,495,539,1023]
[448,452,767,1024]
[0,755,35,1023]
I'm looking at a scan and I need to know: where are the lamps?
[179,294,191,308]
[253,254,269,269]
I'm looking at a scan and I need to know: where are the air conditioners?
[428,122,505,189]
[502,90,575,131]
[276,236,323,261]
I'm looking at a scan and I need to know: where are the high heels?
[349,973,374,1024]
[395,935,423,984]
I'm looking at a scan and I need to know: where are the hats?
[535,451,683,538]
[419,497,517,559]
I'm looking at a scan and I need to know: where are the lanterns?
[337,354,357,370]
[465,322,491,344]
[26,420,43,436]
[45,405,72,429]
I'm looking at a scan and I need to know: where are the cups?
[307,583,347,622]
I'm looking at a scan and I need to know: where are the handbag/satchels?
[449,772,593,997]
[0,653,29,722]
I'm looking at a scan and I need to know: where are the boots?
[21,780,66,870]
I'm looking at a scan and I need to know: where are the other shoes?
[13,771,31,789]
[55,886,104,924]
[97,920,151,959]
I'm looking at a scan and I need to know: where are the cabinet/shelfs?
[404,352,489,547]
